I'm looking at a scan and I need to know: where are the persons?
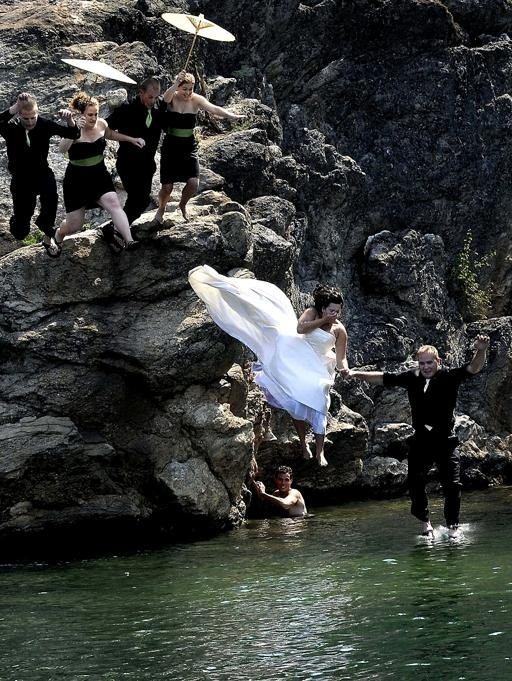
[97,75,171,251]
[190,265,348,465]
[0,93,81,258]
[151,68,247,228]
[251,466,308,517]
[53,93,147,251]
[342,331,490,546]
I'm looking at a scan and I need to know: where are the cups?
[418,361,433,365]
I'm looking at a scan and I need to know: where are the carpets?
[146,109,153,129]
[25,130,30,147]
[423,378,431,393]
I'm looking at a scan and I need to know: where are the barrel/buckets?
[43,240,61,258]
[123,240,139,253]
[55,227,64,248]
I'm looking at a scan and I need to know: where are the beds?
[61,56,137,115]
[160,9,234,83]
